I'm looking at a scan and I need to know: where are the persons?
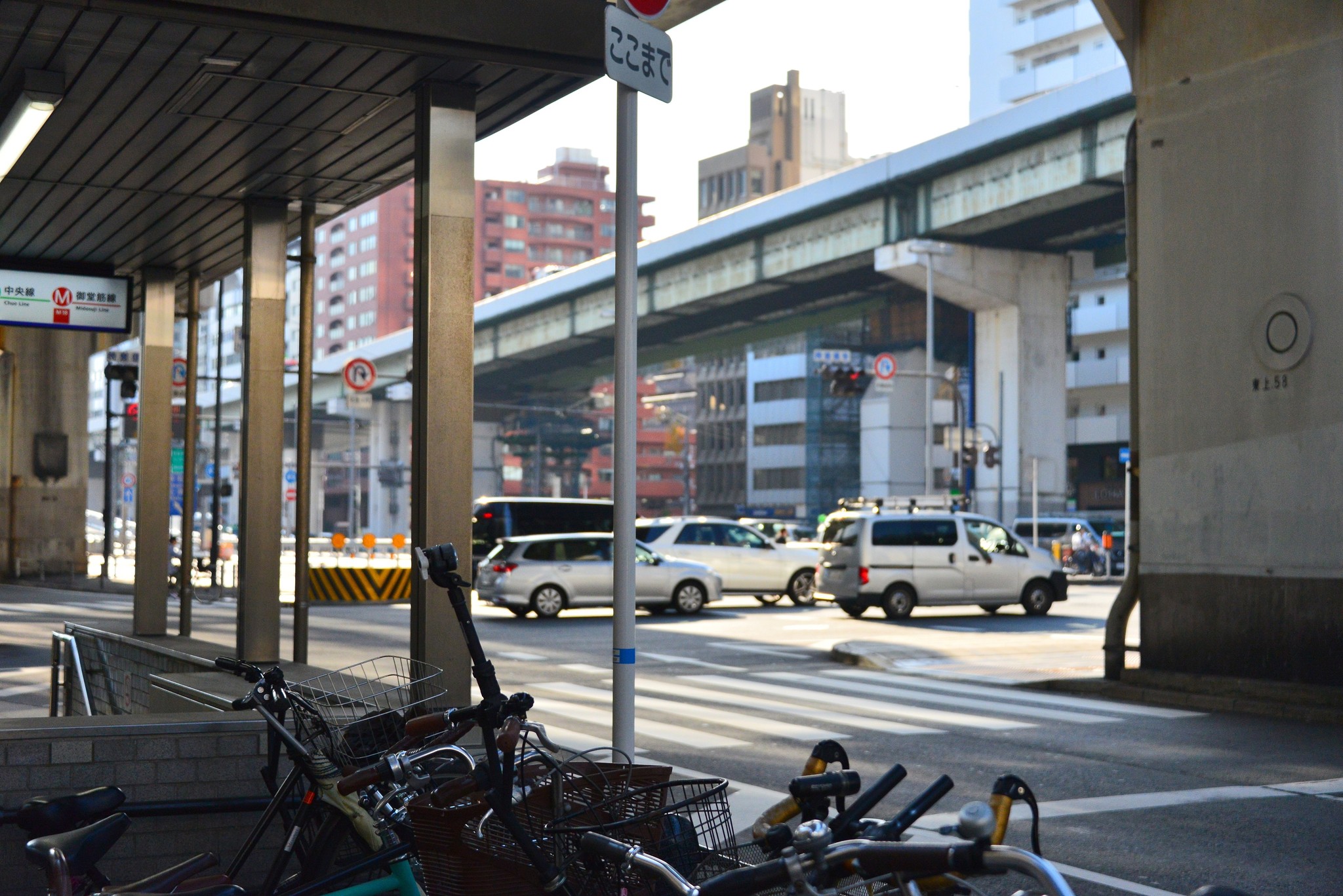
[1071,523,1093,574]
[775,528,788,544]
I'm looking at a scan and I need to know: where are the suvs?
[470,495,615,587]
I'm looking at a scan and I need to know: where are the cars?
[475,530,726,620]
[634,515,824,607]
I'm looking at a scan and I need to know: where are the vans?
[1012,517,1105,554]
[818,493,1070,617]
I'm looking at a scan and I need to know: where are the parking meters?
[1102,530,1114,577]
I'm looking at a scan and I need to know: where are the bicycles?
[1,543,1078,895]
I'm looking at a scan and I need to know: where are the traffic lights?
[820,366,865,381]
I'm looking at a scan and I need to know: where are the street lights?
[910,245,953,492]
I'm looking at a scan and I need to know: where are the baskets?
[280,653,455,779]
[406,744,787,896]
